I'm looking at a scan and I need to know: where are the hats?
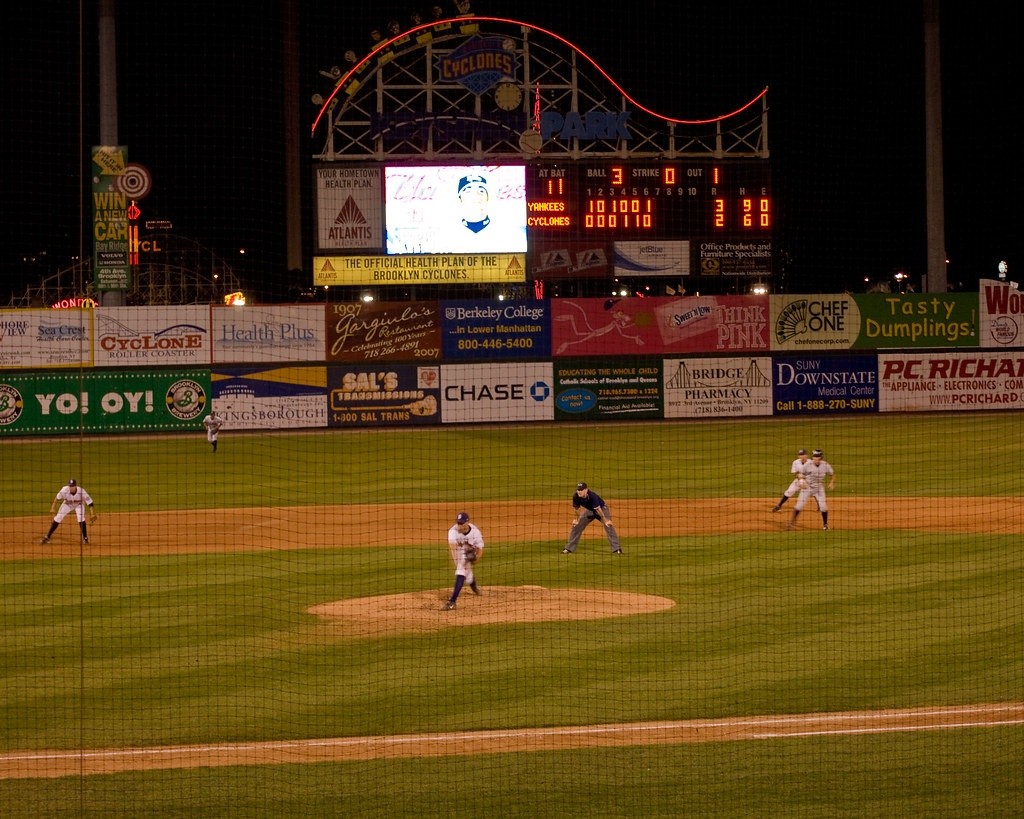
[811,450,823,458]
[210,411,215,414]
[67,479,76,486]
[576,482,587,490]
[456,512,469,523]
[798,450,806,455]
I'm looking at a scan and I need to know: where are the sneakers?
[439,600,456,611]
[473,587,482,596]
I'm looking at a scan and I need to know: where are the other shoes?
[83,537,89,545]
[824,525,829,530]
[40,538,50,544]
[616,549,622,554]
[561,548,570,554]
[772,506,781,512]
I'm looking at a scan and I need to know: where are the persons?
[906,283,914,293]
[772,449,836,531]
[560,482,624,557]
[439,512,484,611]
[40,479,97,545]
[203,411,223,453]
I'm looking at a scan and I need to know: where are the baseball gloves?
[89,515,97,525]
[211,427,218,434]
[463,546,477,562]
